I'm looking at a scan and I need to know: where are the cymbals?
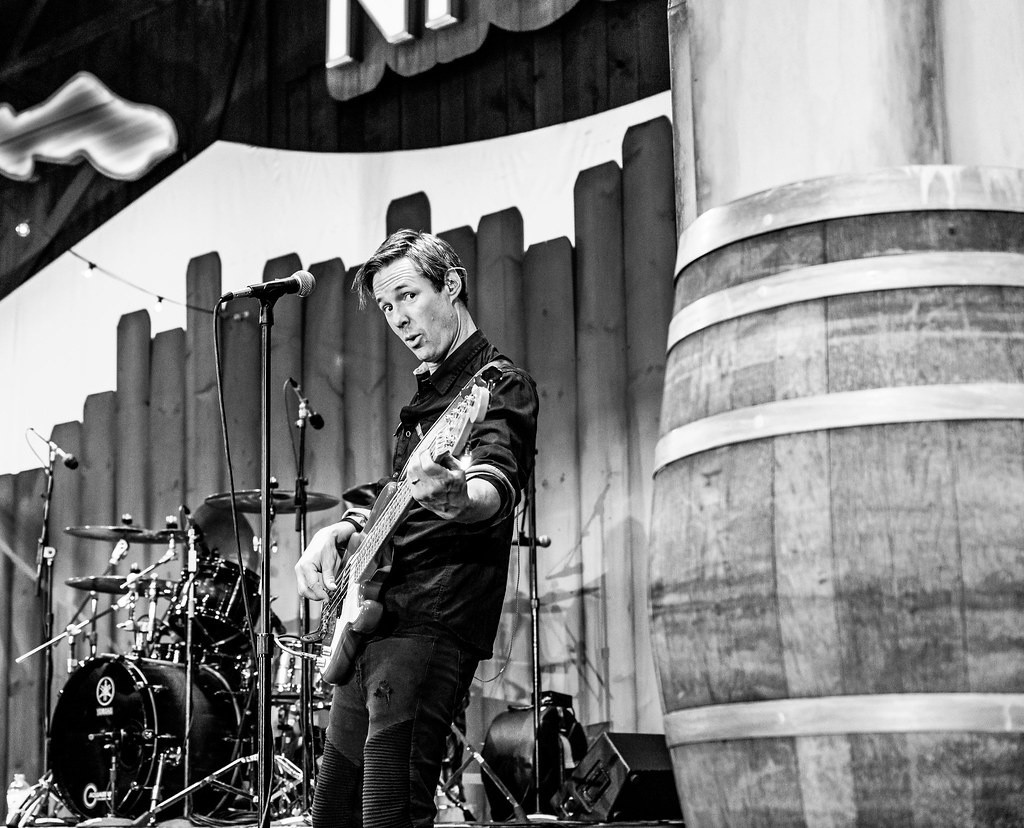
[64,525,185,543]
[63,576,178,595]
[204,489,340,514]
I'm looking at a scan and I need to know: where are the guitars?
[318,365,503,685]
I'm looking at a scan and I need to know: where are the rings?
[412,479,420,484]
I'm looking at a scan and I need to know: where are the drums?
[166,555,262,651]
[140,640,258,698]
[45,653,251,821]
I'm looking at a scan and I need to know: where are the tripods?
[7,451,70,828]
[131,505,317,828]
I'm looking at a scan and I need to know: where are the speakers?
[551,731,684,824]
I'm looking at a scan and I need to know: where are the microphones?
[221,271,316,302]
[32,428,79,470]
[512,535,551,547]
[290,379,324,430]
[183,506,211,557]
[270,610,287,635]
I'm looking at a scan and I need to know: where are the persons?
[294,228,541,828]
